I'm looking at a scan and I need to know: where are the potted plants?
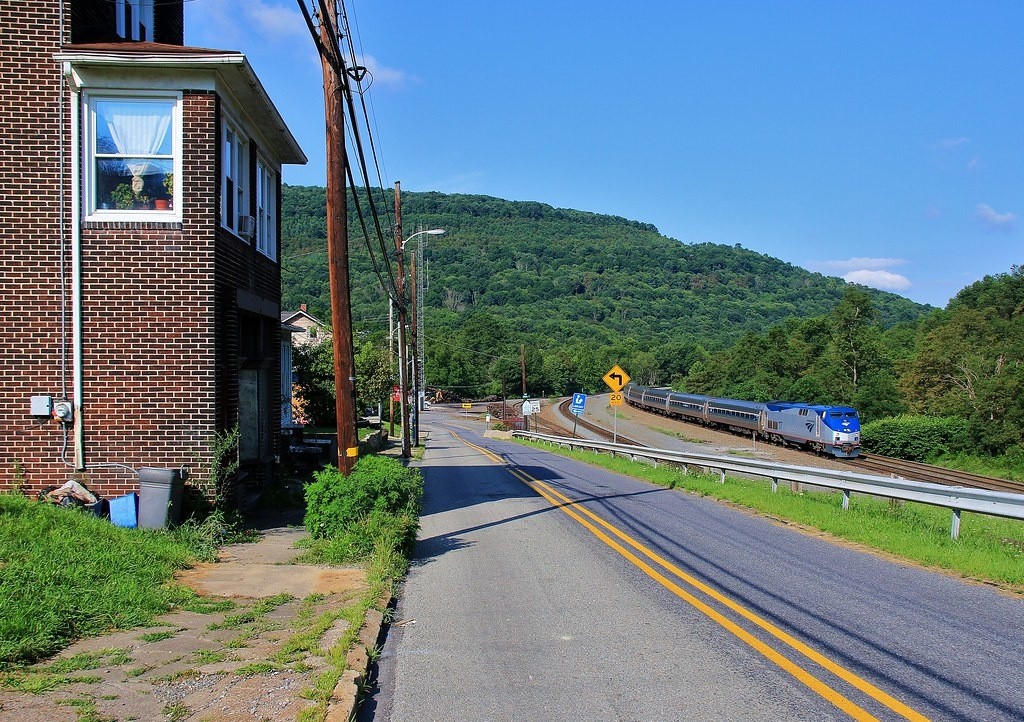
[99,194,110,209]
[134,191,154,208]
[111,183,132,209]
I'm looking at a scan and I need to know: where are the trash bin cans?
[138,467,181,532]
[36,496,105,518]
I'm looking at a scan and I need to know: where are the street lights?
[397,230,447,457]
[390,325,409,436]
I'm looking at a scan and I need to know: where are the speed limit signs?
[530,401,542,414]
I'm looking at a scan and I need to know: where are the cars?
[424,401,431,408]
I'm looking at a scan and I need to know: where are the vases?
[155,200,168,209]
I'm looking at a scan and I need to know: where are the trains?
[624,380,862,458]
